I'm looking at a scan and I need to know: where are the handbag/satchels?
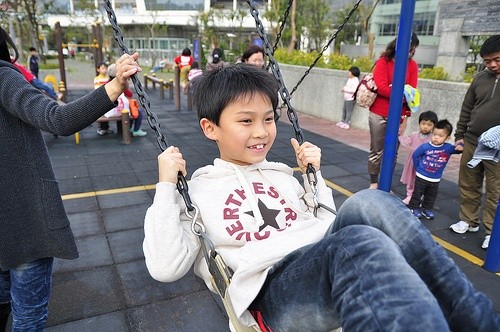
[352,58,380,109]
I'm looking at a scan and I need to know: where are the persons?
[449,34,500,248]
[142,64,500,331]
[149,55,169,74]
[241,44,266,69]
[26,45,40,80]
[69,48,77,58]
[335,66,360,130]
[205,47,231,72]
[174,47,195,95]
[106,63,148,137]
[94,61,114,136]
[62,46,69,59]
[366,31,419,192]
[409,118,466,220]
[398,108,438,206]
[0,26,142,332]
[187,61,204,88]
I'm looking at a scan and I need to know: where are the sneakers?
[449,220,479,234]
[411,208,422,218]
[421,207,434,219]
[132,129,147,136]
[481,235,491,249]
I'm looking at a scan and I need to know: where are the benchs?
[95,110,135,144]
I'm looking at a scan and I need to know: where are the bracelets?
[403,114,408,120]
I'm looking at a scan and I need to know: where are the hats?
[107,64,116,77]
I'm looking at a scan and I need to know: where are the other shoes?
[97,128,113,135]
[336,121,344,127]
[402,196,411,205]
[341,123,350,129]
